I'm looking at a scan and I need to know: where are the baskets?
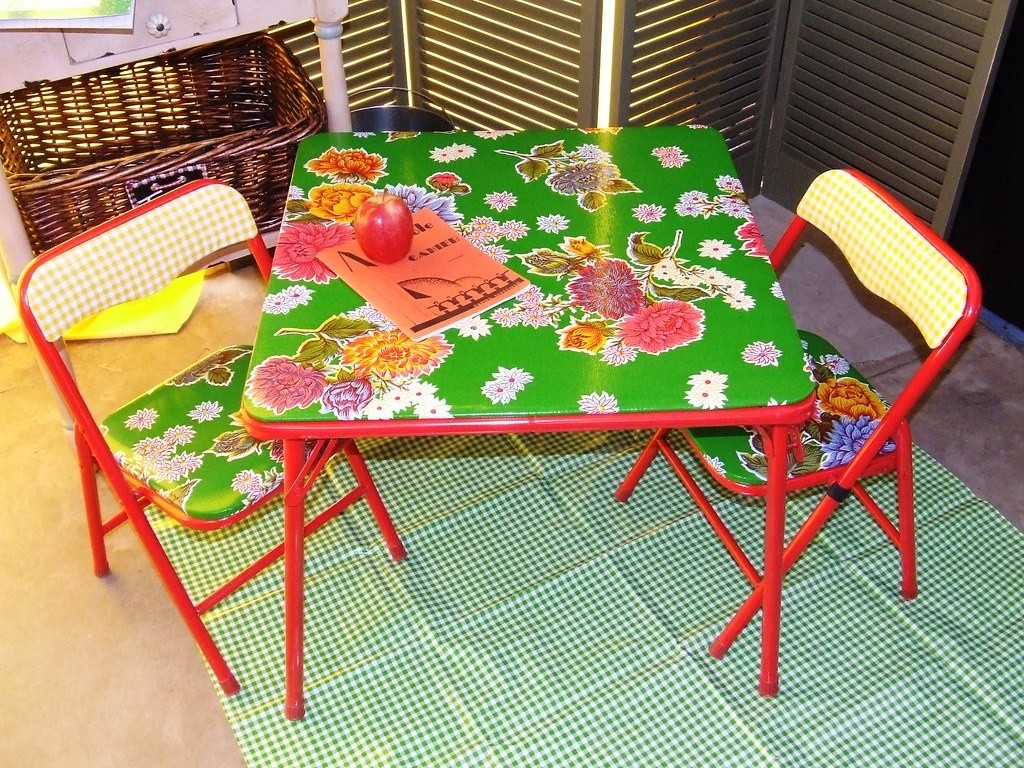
[0,31,327,257]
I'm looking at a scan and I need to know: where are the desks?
[241,124,814,720]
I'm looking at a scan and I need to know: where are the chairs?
[16,180,406,695]
[614,169,983,660]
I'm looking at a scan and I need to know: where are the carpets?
[144,428,1024,768]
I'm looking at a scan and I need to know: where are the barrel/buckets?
[349,86,456,133]
